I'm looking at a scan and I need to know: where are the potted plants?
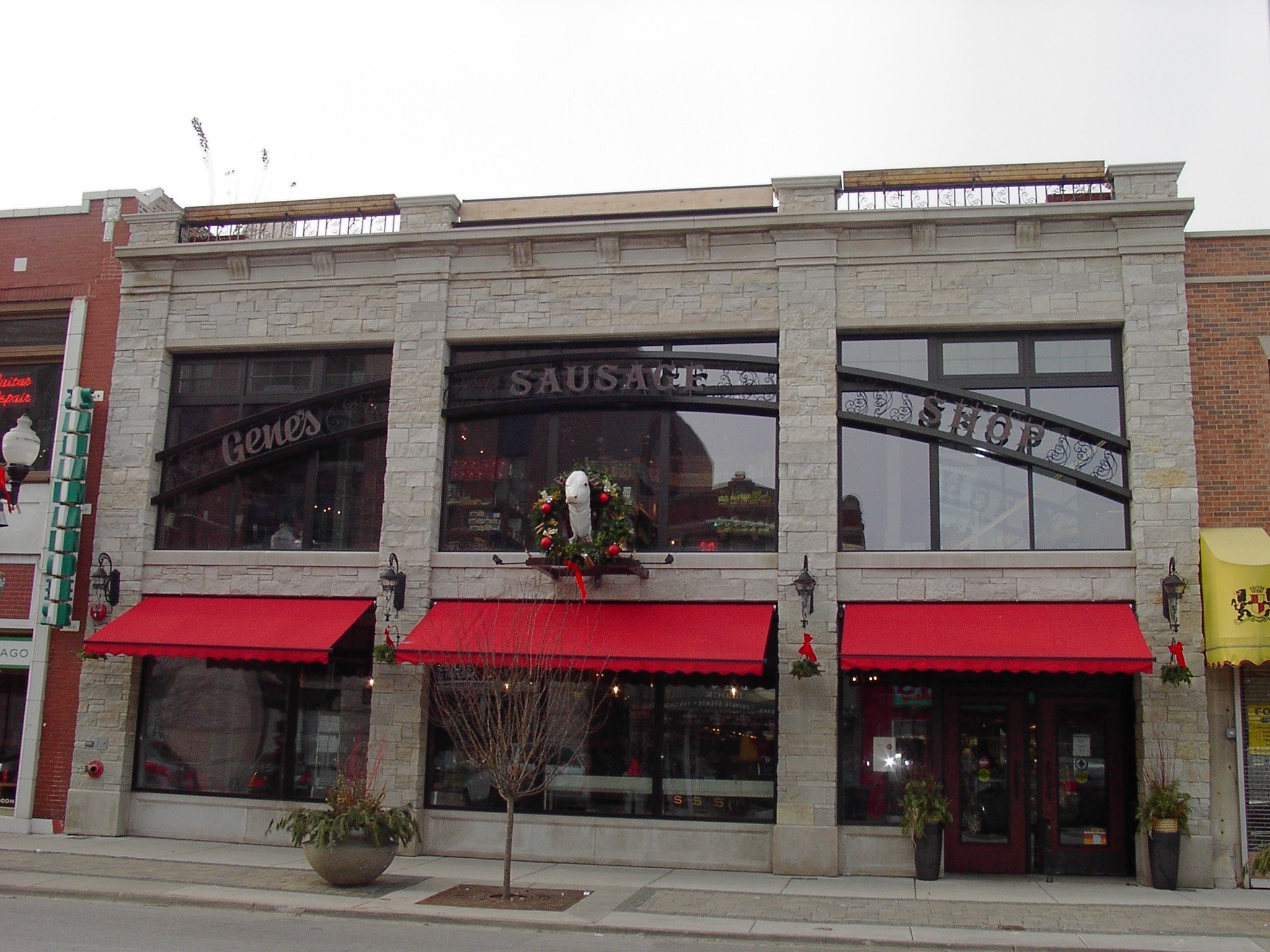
[1136,743,1191,891]
[895,766,953,883]
[264,734,426,892]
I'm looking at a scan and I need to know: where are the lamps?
[1162,558,1190,633]
[378,553,406,622]
[792,555,815,627]
[88,553,120,622]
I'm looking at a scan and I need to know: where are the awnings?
[394,598,776,679]
[1199,527,1270,669]
[79,593,375,663]
[836,600,1159,677]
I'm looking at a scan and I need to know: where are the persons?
[581,715,776,796]
